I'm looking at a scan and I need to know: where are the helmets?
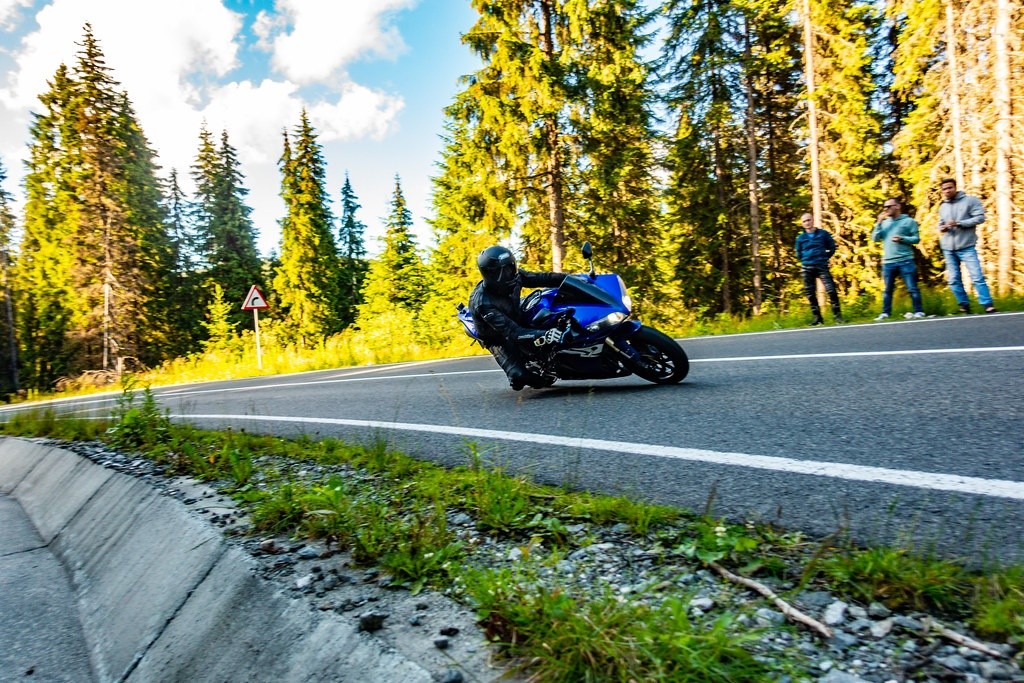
[478,246,520,294]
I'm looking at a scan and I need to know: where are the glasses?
[883,205,896,209]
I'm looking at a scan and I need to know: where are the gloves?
[531,327,563,347]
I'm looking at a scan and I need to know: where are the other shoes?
[873,313,888,321]
[984,303,999,313]
[833,316,848,324]
[951,302,971,315]
[913,312,925,318]
[812,316,823,325]
[526,374,545,388]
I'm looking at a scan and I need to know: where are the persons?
[936,178,1001,315]
[871,198,926,321]
[795,212,850,326]
[468,245,570,391]
